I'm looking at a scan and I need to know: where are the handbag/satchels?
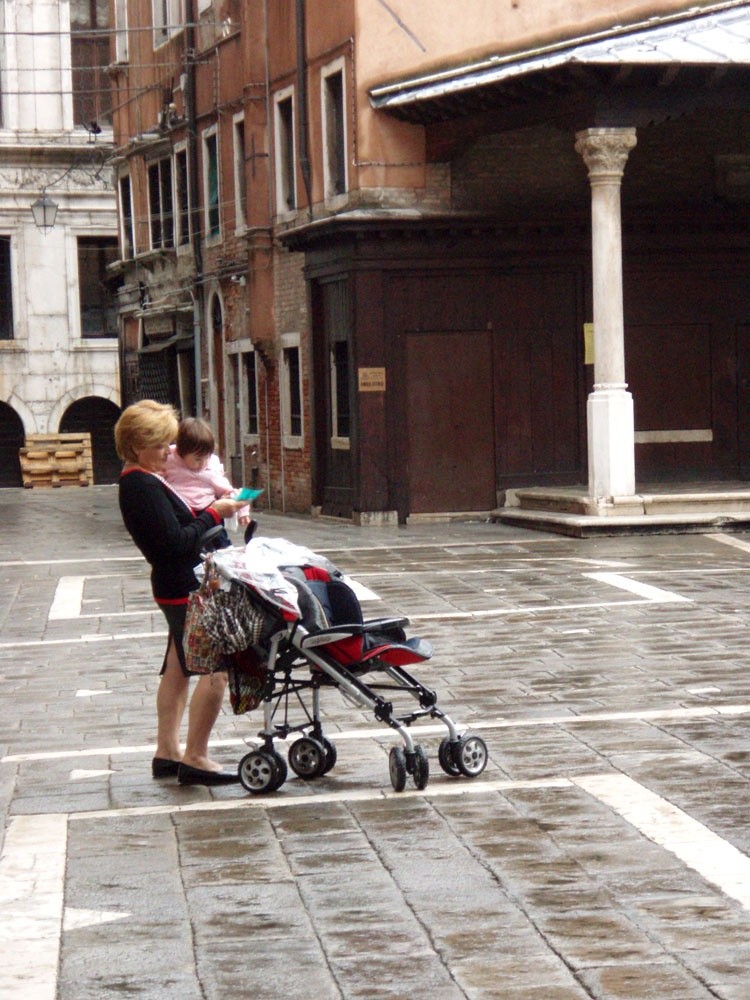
[183,552,224,673]
[226,648,275,715]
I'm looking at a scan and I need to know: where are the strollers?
[195,519,489,794]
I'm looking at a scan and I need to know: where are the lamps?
[31,185,59,236]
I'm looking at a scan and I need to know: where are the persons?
[115,400,251,785]
[152,417,250,552]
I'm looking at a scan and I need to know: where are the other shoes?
[177,762,240,785]
[152,757,180,778]
